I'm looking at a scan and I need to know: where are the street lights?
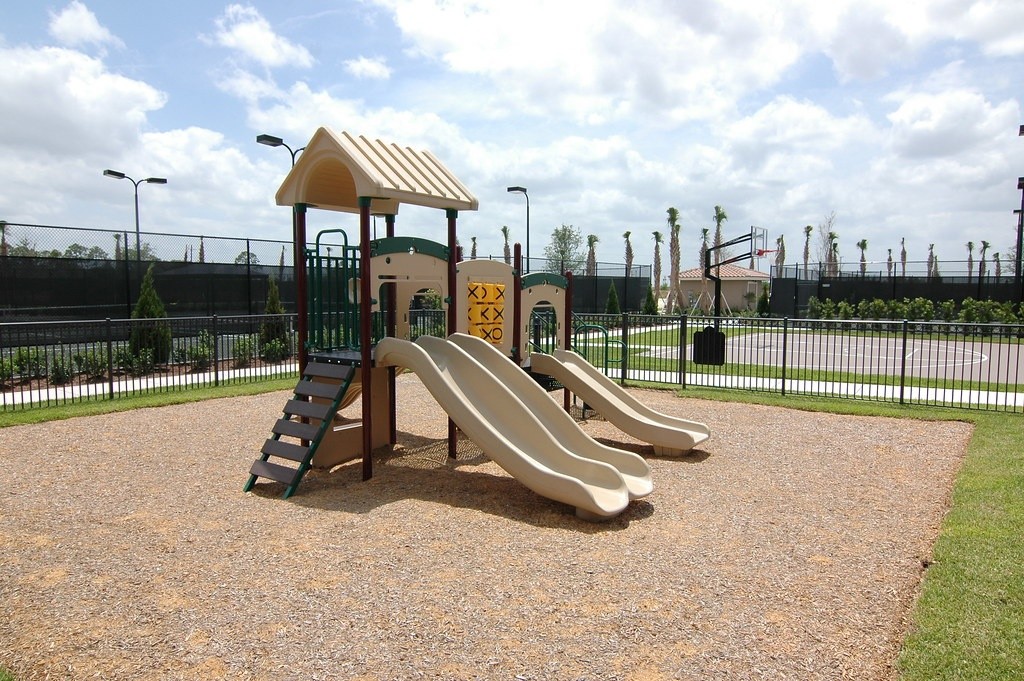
[255,133,308,335]
[507,186,530,274]
[104,169,169,313]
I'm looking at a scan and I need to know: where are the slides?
[529,348,711,449]
[373,331,654,516]
